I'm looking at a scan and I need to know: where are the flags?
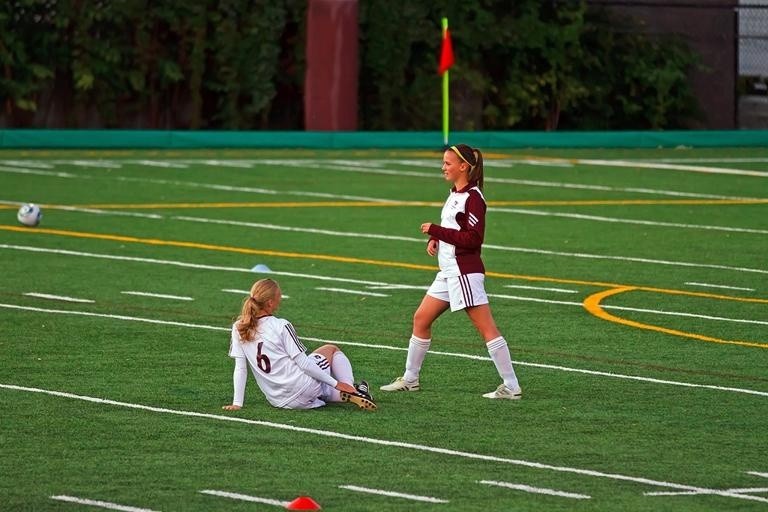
[437,27,456,76]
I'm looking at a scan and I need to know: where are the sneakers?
[380,378,420,393]
[356,380,372,400]
[482,384,522,401]
[340,391,377,412]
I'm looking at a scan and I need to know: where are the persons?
[380,142,522,401]
[218,278,379,412]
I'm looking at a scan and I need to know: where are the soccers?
[18,204,39,226]
